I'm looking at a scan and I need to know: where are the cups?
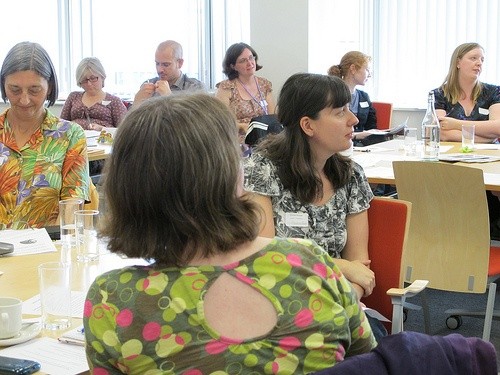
[0,297,23,339]
[59,199,83,248]
[404,128,417,157]
[461,124,476,153]
[74,210,100,264]
[39,262,73,330]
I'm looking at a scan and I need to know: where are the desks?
[350,135,500,191]
[0,240,156,375]
[88,128,119,161]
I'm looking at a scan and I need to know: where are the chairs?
[361,161,500,342]
[363,102,404,146]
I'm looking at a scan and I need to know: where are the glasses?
[237,56,255,65]
[80,76,99,83]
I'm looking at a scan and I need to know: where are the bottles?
[421,91,440,161]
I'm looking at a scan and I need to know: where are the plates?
[0,320,42,346]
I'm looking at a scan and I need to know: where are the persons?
[0,42,92,231]
[241,73,376,339]
[84,90,379,375]
[59,57,130,130]
[327,51,377,132]
[214,43,277,144]
[427,43,500,144]
[133,41,204,107]
